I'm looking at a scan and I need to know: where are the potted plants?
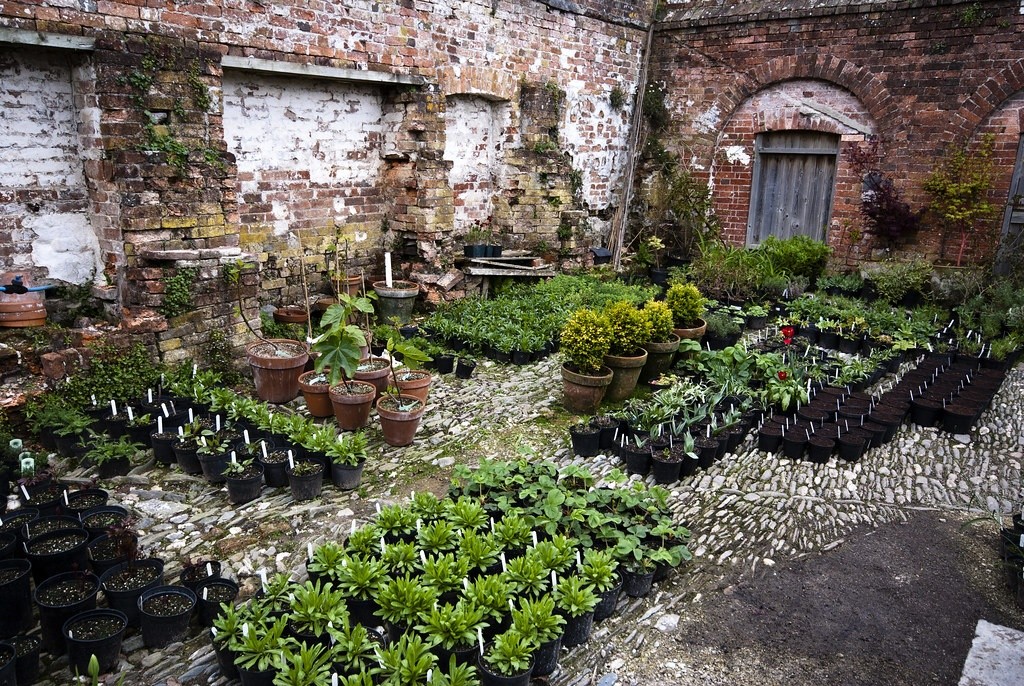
[0,226,1024,686]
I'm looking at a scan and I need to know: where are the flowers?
[763,369,808,410]
[776,324,804,384]
